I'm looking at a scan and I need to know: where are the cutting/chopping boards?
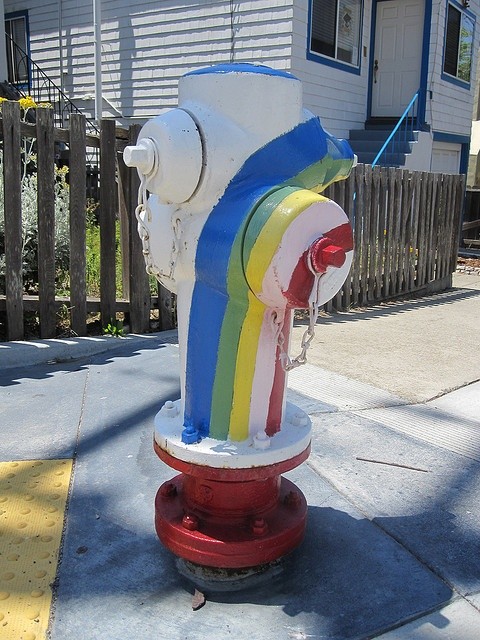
[125,63,360,594]
